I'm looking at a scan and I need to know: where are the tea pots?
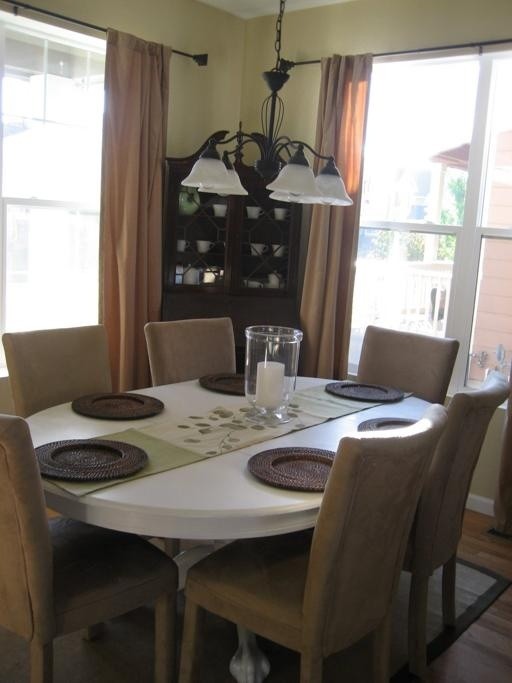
[185,264,203,283]
[268,269,283,287]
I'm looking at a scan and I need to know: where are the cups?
[197,241,215,251]
[256,361,284,409]
[272,243,290,256]
[248,207,259,217]
[274,208,291,219]
[244,280,264,288]
[245,327,301,423]
[249,243,269,255]
[213,204,226,215]
[176,238,189,252]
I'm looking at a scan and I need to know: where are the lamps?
[180,0,354,207]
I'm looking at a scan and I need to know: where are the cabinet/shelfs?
[158,129,304,379]
